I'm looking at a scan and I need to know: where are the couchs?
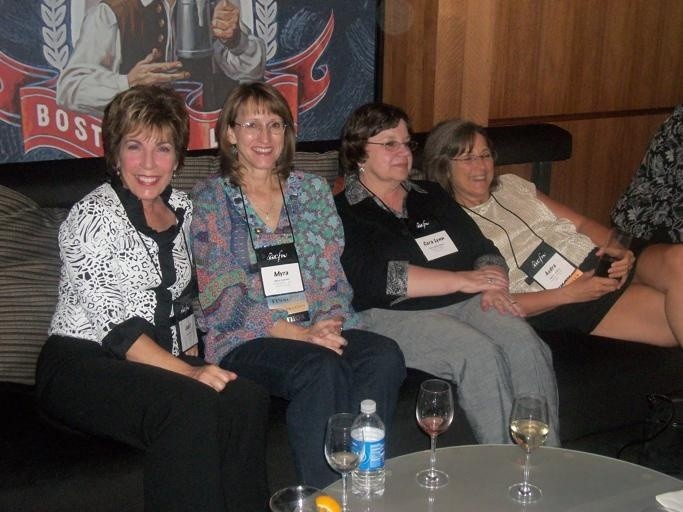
[0,125,683,512]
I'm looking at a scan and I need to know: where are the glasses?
[233,118,288,132]
[451,151,492,164]
[365,140,419,151]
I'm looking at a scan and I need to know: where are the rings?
[489,277,494,286]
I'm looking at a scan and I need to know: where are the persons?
[608,101,683,245]
[424,117,683,350]
[188,82,405,495]
[334,102,561,447]
[36,84,270,512]
[55,1,266,115]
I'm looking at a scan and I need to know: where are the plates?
[268,485,330,512]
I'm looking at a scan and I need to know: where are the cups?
[416,379,455,490]
[595,228,632,278]
[507,393,550,506]
[324,413,366,512]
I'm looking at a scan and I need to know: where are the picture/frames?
[0,1,384,175]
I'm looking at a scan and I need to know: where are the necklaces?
[237,176,281,220]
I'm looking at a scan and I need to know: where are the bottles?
[350,400,385,498]
